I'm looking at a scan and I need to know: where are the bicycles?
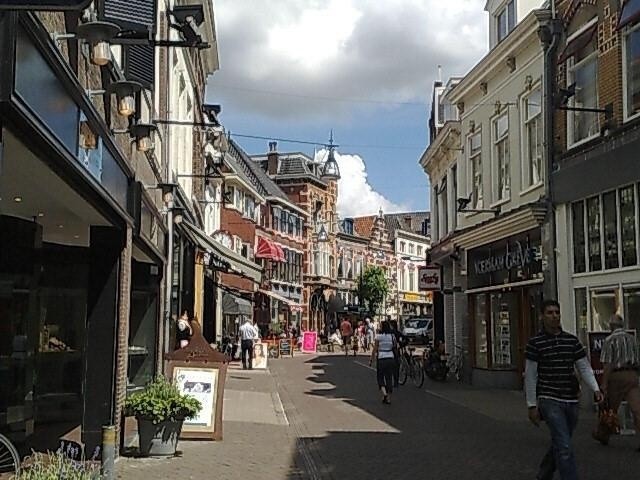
[0,435,20,480]
[422,343,464,382]
[395,342,425,388]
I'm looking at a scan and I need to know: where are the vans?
[402,316,434,345]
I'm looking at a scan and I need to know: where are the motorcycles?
[220,328,242,364]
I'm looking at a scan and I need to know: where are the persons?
[592,315,640,455]
[524,300,604,480]
[320,314,410,405]
[253,346,265,369]
[184,382,209,393]
[237,318,257,370]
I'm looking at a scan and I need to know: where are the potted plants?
[119,374,204,456]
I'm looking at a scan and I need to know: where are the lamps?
[545,85,614,121]
[57,19,124,62]
[87,76,141,117]
[110,123,158,152]
[455,195,502,216]
[143,177,182,202]
[157,206,186,228]
